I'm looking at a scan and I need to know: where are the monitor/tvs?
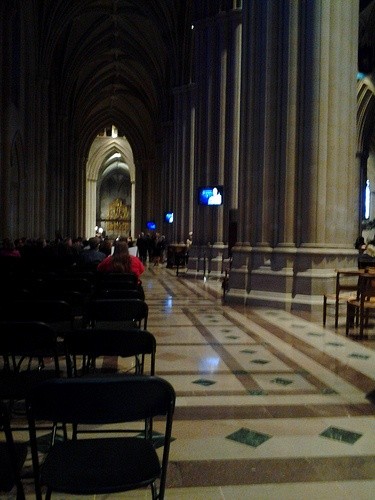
[164,211,174,224]
[197,184,225,207]
[144,220,157,230]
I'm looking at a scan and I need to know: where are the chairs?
[323,271,375,339]
[0,255,186,500]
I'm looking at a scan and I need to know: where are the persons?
[0,230,144,328]
[355,235,375,269]
[136,232,167,266]
[184,234,192,264]
